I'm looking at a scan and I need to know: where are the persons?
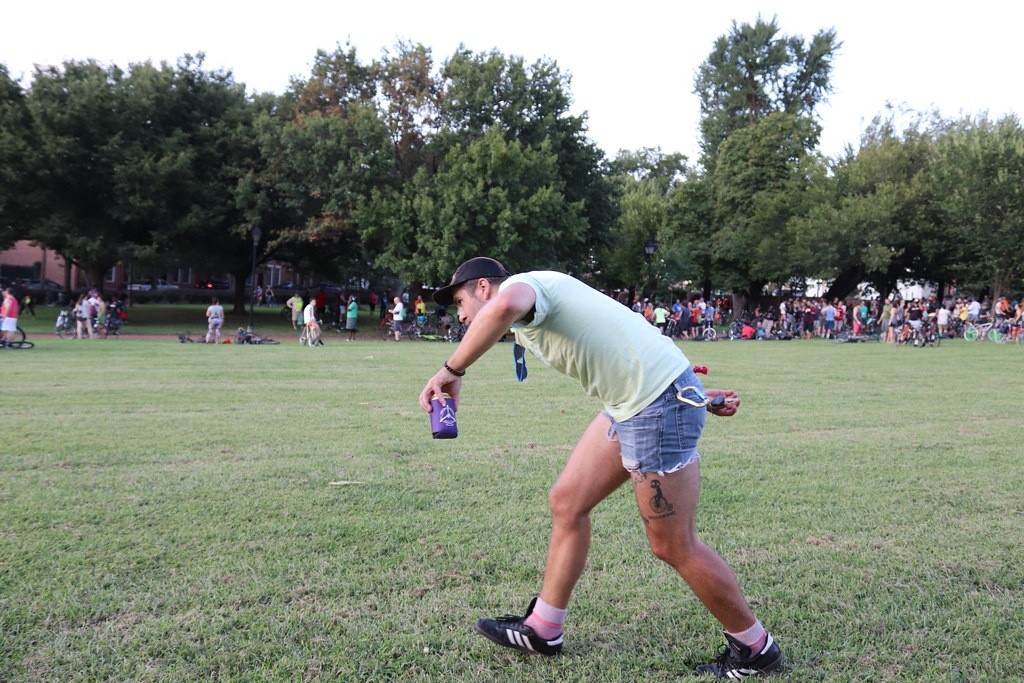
[627,293,1024,344]
[286,286,451,347]
[419,257,782,678]
[205,297,224,343]
[0,276,34,346]
[54,284,130,339]
[252,284,275,307]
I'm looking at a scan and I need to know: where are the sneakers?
[695,632,782,680]
[474,597,562,657]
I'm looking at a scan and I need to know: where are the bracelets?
[444,362,465,376]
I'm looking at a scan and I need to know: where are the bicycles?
[0,325,34,349]
[703,317,716,339]
[964,314,1002,342]
[57,304,109,339]
[281,306,292,321]
[995,317,1024,346]
[242,332,280,344]
[299,322,321,347]
[447,322,468,342]
[898,323,940,347]
[381,311,454,340]
[175,331,215,343]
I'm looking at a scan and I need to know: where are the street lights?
[248,226,262,333]
[643,240,655,300]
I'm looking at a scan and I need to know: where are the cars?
[128,278,179,292]
[273,281,305,290]
[196,279,231,289]
[772,287,805,298]
[318,281,360,290]
[11,279,65,304]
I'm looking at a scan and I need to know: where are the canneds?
[428,392,458,439]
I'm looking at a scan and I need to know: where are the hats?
[928,293,937,298]
[434,257,512,305]
